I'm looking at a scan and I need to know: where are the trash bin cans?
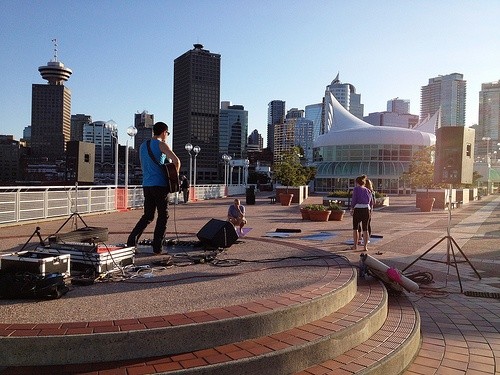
[246,188,255,205]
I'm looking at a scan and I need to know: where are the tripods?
[401,185,482,293]
[55,181,88,234]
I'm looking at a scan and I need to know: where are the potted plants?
[300,204,345,221]
[417,171,436,212]
[330,190,390,208]
[278,166,295,206]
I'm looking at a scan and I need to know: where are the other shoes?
[127,242,137,250]
[155,250,168,256]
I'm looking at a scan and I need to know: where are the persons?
[127,122,181,255]
[358,179,373,240]
[351,175,372,250]
[227,198,247,233]
[180,175,189,203]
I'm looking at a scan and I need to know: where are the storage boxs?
[36,244,135,278]
[0,251,71,279]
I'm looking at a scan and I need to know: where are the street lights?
[222,153,232,195]
[185,142,200,203]
[242,158,250,192]
[125,125,137,207]
[107,120,120,209]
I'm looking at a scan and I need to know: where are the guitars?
[163,162,180,193]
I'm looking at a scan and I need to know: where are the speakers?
[66,140,96,183]
[196,218,239,249]
[433,125,476,184]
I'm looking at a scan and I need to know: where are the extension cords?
[194,252,218,263]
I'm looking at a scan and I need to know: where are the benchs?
[447,201,459,208]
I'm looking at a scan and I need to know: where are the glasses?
[166,131,169,135]
